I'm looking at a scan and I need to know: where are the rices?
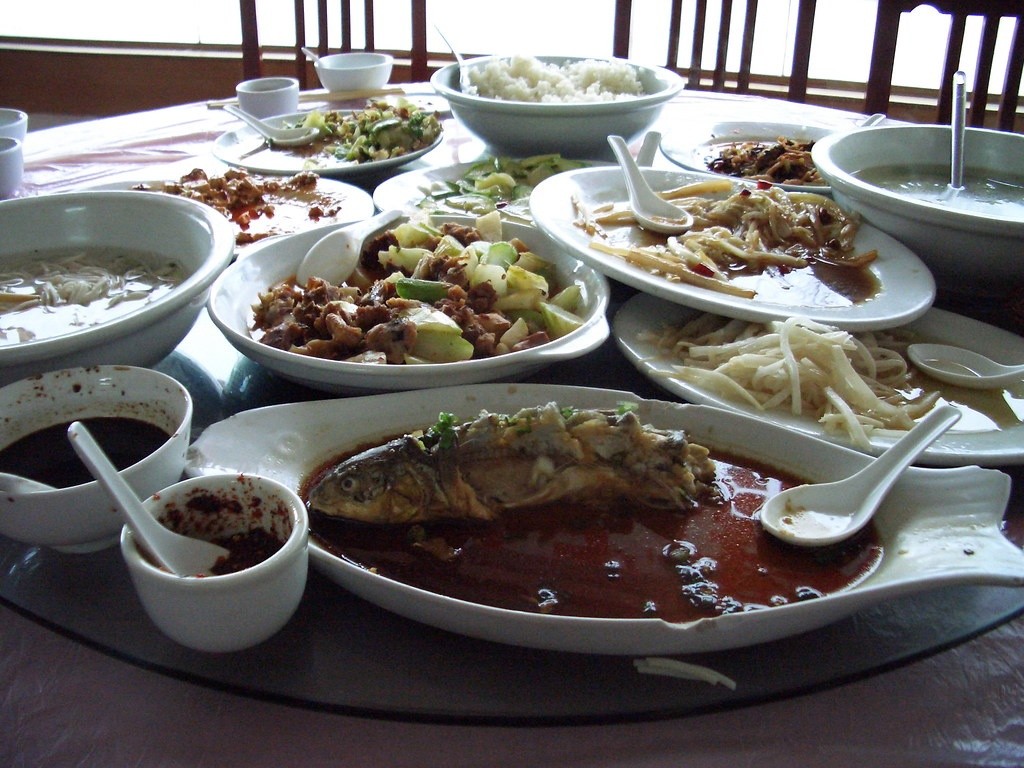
[464,53,650,103]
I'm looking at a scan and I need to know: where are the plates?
[204,215,613,395]
[180,381,1024,659]
[611,280,1024,473]
[529,164,938,330]
[370,147,622,229]
[658,119,850,198]
[209,110,443,174]
[65,171,376,261]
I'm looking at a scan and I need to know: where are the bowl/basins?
[314,52,394,94]
[0,137,23,198]
[0,364,192,555]
[811,123,1024,309]
[0,188,235,387]
[427,54,686,158]
[0,106,27,152]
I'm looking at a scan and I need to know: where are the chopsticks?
[206,88,406,110]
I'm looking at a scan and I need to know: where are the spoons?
[607,133,695,235]
[905,342,1024,390]
[762,404,963,547]
[222,104,319,146]
[296,211,402,286]
[67,422,229,578]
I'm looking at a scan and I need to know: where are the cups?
[121,472,308,654]
[235,77,299,122]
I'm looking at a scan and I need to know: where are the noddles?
[0,245,186,349]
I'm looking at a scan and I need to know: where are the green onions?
[428,401,639,449]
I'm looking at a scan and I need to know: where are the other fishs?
[305,398,715,527]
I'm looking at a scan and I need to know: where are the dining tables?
[0,82,1024,768]
